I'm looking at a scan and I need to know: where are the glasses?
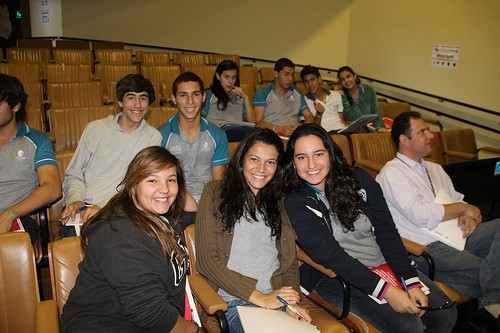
[418,297,457,313]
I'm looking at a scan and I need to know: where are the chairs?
[0,46,500,333]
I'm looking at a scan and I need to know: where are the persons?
[202,60,254,142]
[253,58,314,135]
[157,71,229,202]
[286,123,458,333]
[337,66,388,132]
[61,146,198,333]
[0,73,62,234]
[59,74,163,237]
[195,128,323,333]
[376,112,500,304]
[299,65,350,132]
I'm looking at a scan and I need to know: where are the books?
[10,218,25,232]
[368,263,431,305]
[66,205,93,226]
[338,114,379,133]
[420,190,466,251]
[368,117,394,132]
[236,306,320,333]
[220,122,257,127]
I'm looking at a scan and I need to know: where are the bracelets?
[273,125,275,130]
[4,208,16,220]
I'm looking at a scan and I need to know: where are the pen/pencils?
[58,206,87,220]
[401,275,410,299]
[276,294,306,319]
